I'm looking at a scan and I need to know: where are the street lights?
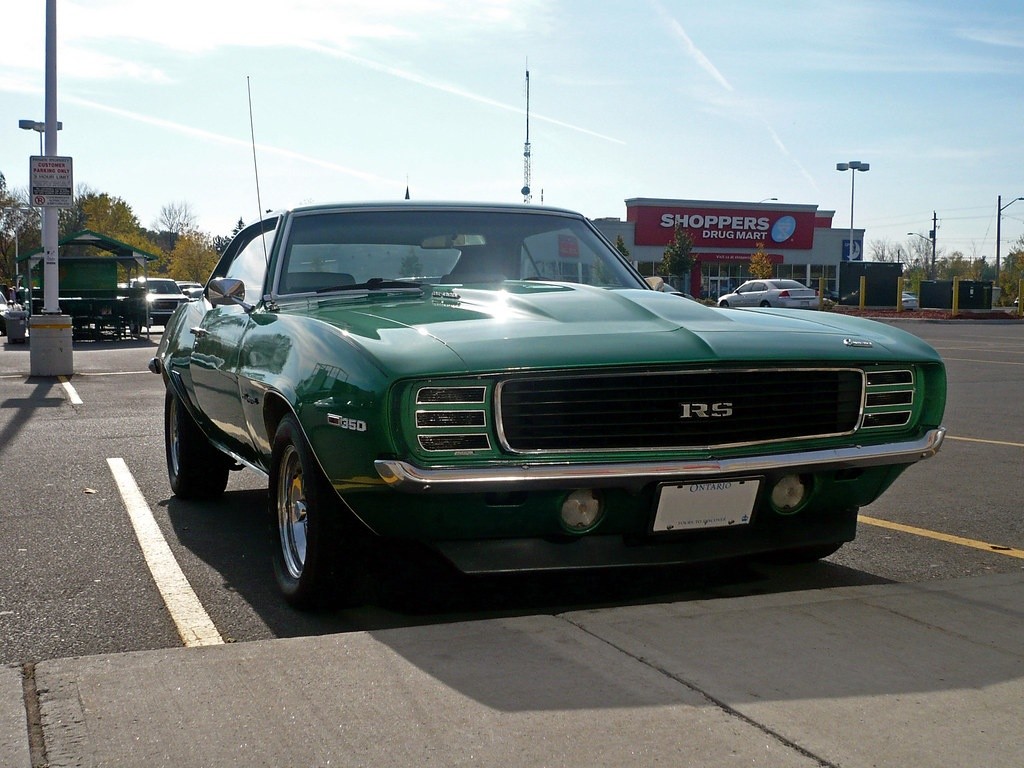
[995,194,1024,287]
[907,210,937,280]
[836,161,869,261]
[17,120,63,156]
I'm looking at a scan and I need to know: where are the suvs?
[126,278,189,334]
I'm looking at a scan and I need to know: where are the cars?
[717,279,822,311]
[147,200,949,618]
[175,279,205,302]
[117,281,131,303]
[822,290,838,304]
[901,289,919,312]
[0,291,16,336]
[652,282,696,301]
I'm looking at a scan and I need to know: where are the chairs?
[440,272,506,283]
[286,272,357,294]
[75,301,125,344]
